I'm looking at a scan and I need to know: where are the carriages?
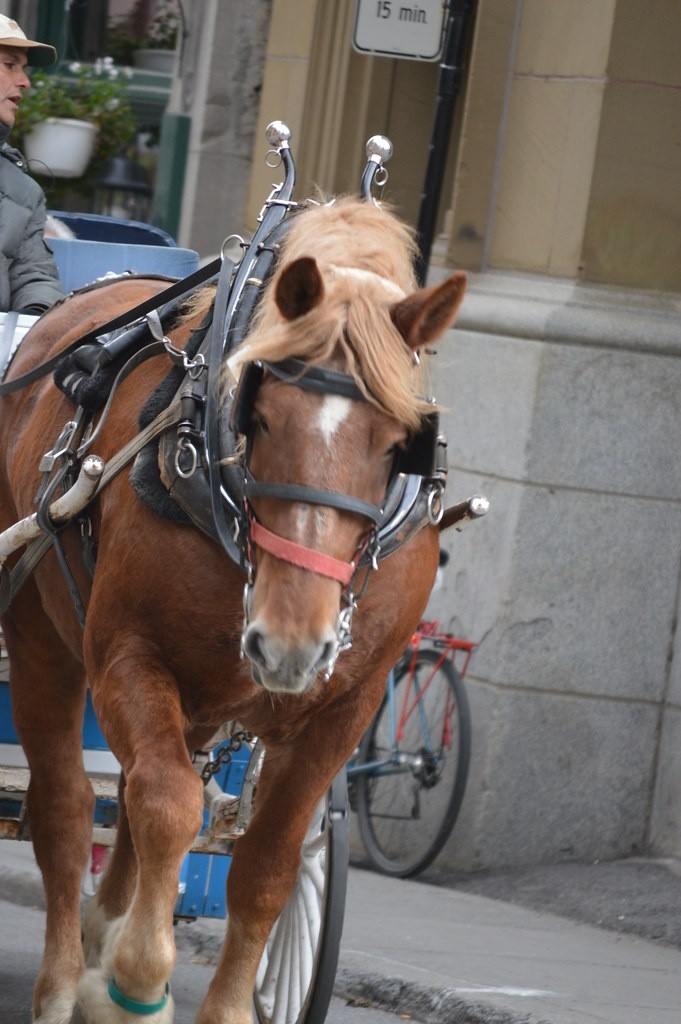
[0,120,489,1024]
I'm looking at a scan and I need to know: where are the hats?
[0,14,57,68]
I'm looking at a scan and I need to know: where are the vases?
[22,116,101,178]
[131,47,175,73]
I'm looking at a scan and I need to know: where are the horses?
[0,191,470,1024]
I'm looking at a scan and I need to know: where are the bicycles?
[347,618,478,881]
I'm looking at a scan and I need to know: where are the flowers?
[10,55,157,170]
[102,0,181,66]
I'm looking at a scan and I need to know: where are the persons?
[0,10,69,318]
